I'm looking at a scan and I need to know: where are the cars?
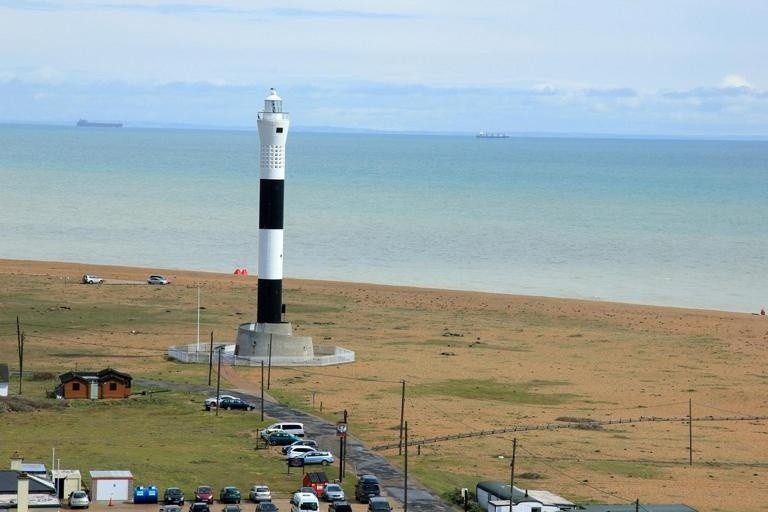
[158,474,392,512]
[261,420,333,468]
[68,488,89,508]
[204,395,241,408]
[147,277,167,285]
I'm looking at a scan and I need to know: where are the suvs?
[148,274,171,285]
[83,274,104,284]
[219,398,256,412]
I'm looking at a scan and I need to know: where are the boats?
[75,119,123,129]
[475,132,511,139]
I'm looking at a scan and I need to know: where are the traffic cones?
[108,497,114,506]
